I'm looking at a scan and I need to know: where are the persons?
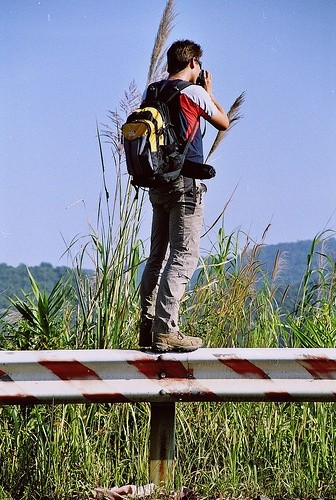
[136,41,228,353]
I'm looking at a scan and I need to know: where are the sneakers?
[152,331,202,352]
[138,331,152,347]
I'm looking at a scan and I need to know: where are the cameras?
[197,70,206,84]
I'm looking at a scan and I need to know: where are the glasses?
[189,58,202,69]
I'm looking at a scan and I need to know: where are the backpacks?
[122,82,196,187]
